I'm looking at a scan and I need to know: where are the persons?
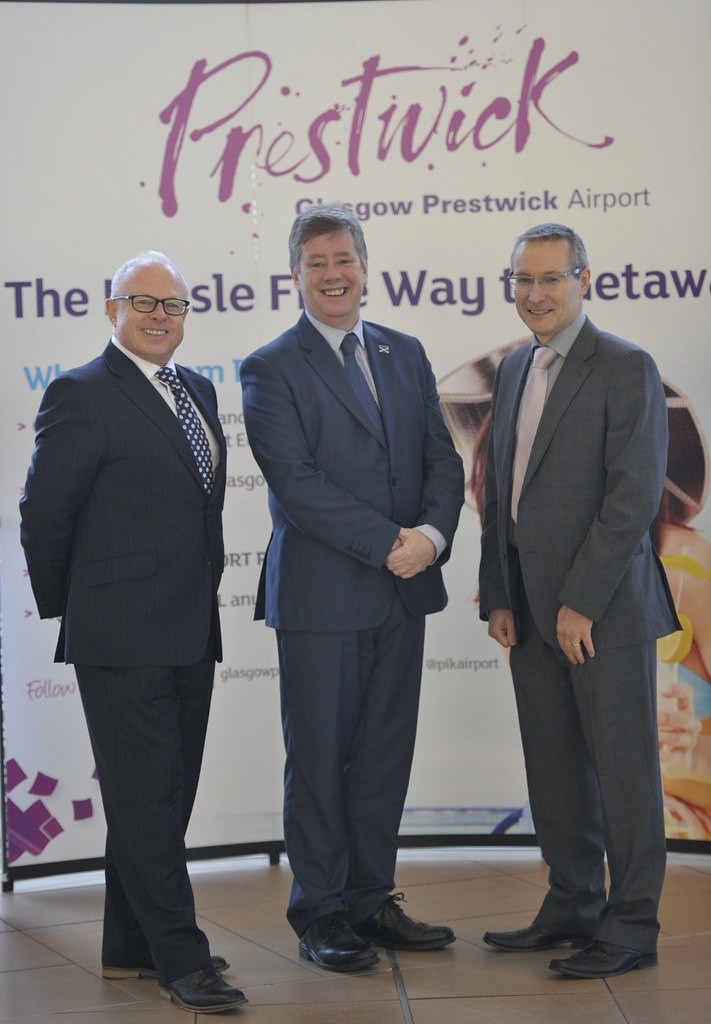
[238,201,465,970]
[437,333,711,841]
[478,223,683,979]
[18,250,248,1012]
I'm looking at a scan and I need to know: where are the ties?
[154,366,215,493]
[510,347,558,524]
[339,332,384,446]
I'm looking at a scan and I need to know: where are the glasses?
[508,267,586,291]
[112,294,190,316]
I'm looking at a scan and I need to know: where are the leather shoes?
[351,893,456,950]
[158,964,248,1010]
[297,910,380,971]
[482,922,596,951]
[549,941,657,979]
[102,956,231,979]
